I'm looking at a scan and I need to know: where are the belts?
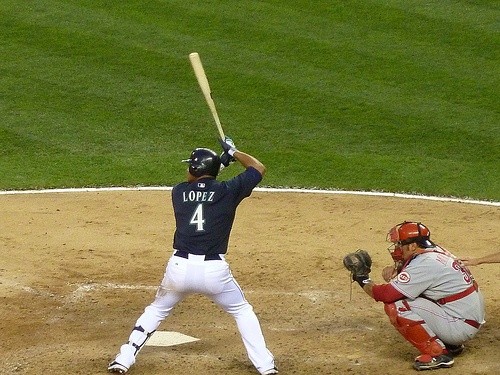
[465,319,483,330]
[174,250,222,260]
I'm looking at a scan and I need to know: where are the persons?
[342,221,486,370]
[459,249,500,266]
[107,135,278,375]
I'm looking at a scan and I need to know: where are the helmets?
[182,148,220,177]
[387,221,431,243]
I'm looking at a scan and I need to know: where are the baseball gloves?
[344,250,372,278]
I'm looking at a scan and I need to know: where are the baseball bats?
[189,52,225,140]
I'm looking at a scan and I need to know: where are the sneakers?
[446,343,464,356]
[263,366,279,375]
[412,354,454,370]
[108,360,128,375]
